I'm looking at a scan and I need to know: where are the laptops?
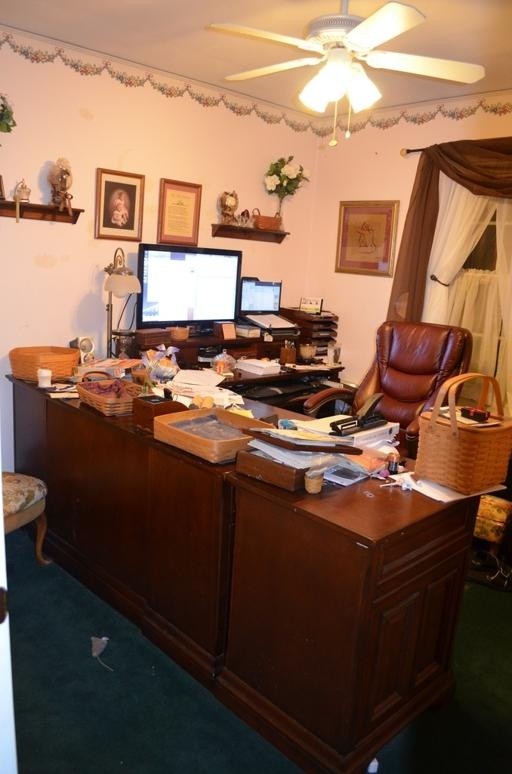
[240,277,300,330]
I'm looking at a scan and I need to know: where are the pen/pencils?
[285,340,294,348]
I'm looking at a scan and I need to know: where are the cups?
[304,470,324,494]
[38,369,52,388]
[328,341,342,366]
[130,366,146,385]
[300,343,315,364]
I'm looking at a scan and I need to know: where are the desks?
[4,371,509,774]
[142,331,344,419]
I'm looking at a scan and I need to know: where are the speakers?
[68,336,96,362]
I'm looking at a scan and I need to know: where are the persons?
[110,191,130,225]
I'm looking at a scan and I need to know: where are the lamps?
[295,54,382,146]
[104,248,142,357]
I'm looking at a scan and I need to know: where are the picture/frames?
[94,168,145,242]
[335,201,400,277]
[157,178,203,247]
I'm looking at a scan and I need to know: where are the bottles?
[211,349,237,374]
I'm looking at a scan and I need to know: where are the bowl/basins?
[168,327,188,341]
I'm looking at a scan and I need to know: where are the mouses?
[304,359,311,364]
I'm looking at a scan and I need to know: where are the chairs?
[3,465,52,566]
[302,321,473,460]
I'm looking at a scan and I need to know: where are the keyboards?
[247,384,313,398]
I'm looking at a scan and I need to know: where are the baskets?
[78,371,149,416]
[9,346,79,381]
[415,373,512,495]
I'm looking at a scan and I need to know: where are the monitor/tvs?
[136,244,242,335]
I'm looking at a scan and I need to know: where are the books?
[240,413,401,487]
[429,405,502,427]
[238,358,281,376]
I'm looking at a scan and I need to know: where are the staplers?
[329,393,388,437]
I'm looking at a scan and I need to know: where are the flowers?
[0,92,17,133]
[264,155,312,217]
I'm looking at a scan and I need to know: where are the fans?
[206,0,486,146]
